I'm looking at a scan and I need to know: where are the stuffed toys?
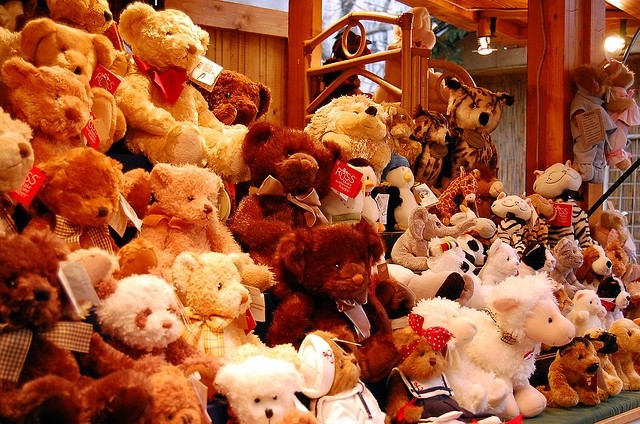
[0,102,35,235]
[0,229,155,424]
[372,181,404,232]
[194,70,271,126]
[422,237,490,311]
[608,319,640,391]
[490,190,533,259]
[479,240,519,286]
[477,164,504,219]
[519,240,556,277]
[140,366,211,424]
[411,296,510,415]
[591,200,640,280]
[540,280,574,351]
[604,229,635,286]
[522,191,553,246]
[320,158,388,232]
[267,219,399,384]
[450,205,498,276]
[18,17,114,81]
[306,95,390,188]
[22,148,126,251]
[385,313,503,424]
[565,289,606,338]
[466,271,575,422]
[574,244,613,291]
[536,337,600,407]
[90,275,219,404]
[312,30,372,104]
[374,5,450,106]
[298,331,387,423]
[213,357,319,424]
[234,120,400,313]
[566,63,616,186]
[424,166,479,226]
[137,164,277,293]
[390,205,477,270]
[552,236,585,301]
[382,148,419,231]
[0,0,133,77]
[410,105,458,199]
[595,56,640,172]
[171,252,299,366]
[114,3,249,184]
[1,58,116,167]
[381,100,422,163]
[597,273,632,333]
[583,327,623,402]
[442,74,515,183]
[532,158,602,249]
